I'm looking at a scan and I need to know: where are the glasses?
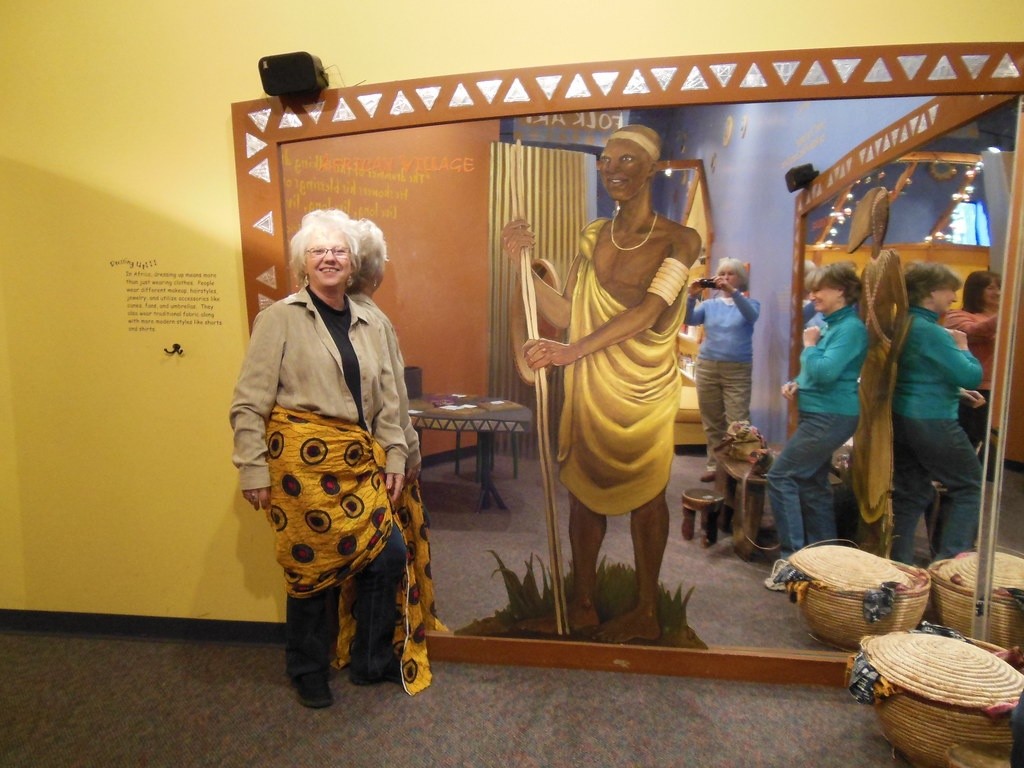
[305,246,350,259]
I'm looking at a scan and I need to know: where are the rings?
[251,494,255,499]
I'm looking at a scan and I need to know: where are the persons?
[946,270,1002,450]
[233,208,433,707]
[764,263,870,590]
[894,264,983,564]
[804,261,827,331]
[352,218,448,632]
[503,125,700,644]
[684,257,759,480]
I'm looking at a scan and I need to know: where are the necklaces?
[610,209,658,251]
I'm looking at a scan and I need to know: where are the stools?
[682,488,724,546]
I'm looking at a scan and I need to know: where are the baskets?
[857,634,1024,765]
[787,545,932,652]
[922,548,1024,652]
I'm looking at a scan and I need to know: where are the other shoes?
[350,654,403,686]
[765,577,786,590]
[700,469,717,483]
[291,670,334,707]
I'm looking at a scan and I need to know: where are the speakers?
[785,164,818,192]
[258,52,330,96]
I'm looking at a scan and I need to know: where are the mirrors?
[785,91,1024,564]
[232,42,1024,686]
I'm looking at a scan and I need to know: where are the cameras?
[700,279,716,289]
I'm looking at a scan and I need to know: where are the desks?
[716,440,852,562]
[409,391,532,511]
